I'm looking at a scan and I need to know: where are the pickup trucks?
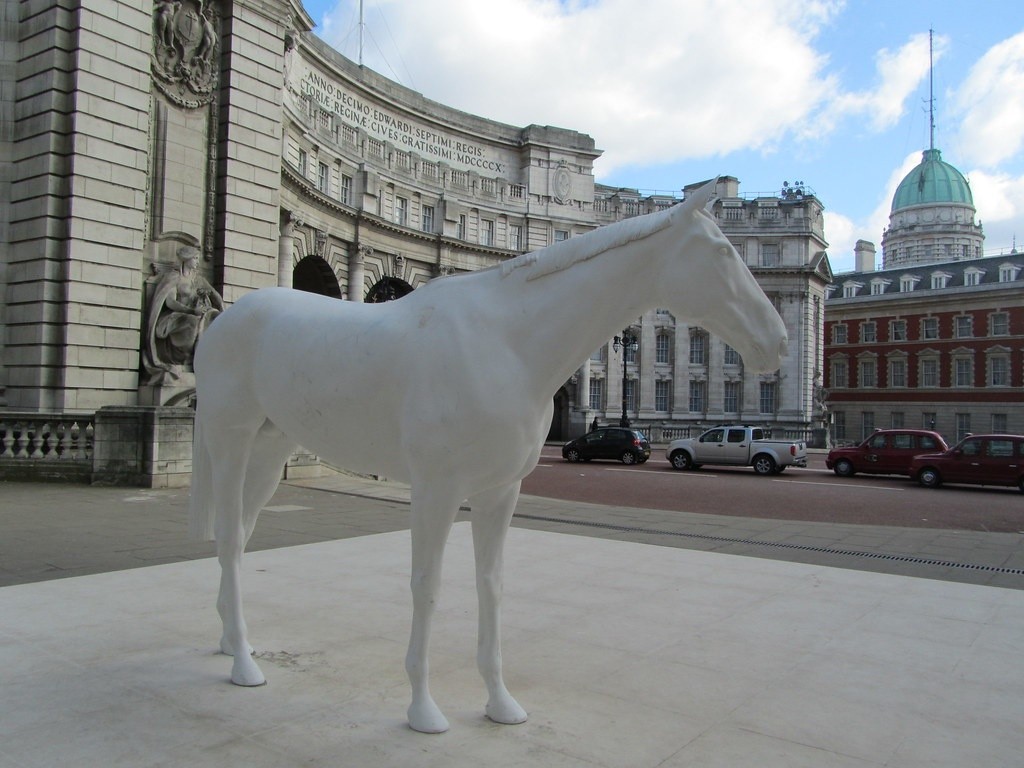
[666,424,809,475]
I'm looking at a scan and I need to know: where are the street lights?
[613,329,638,427]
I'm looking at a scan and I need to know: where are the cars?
[562,427,651,464]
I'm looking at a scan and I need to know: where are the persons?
[813,371,824,403]
[592,417,598,432]
[153,0,182,52]
[143,245,225,386]
[198,0,219,66]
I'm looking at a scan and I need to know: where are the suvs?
[909,432,1024,490]
[826,427,948,477]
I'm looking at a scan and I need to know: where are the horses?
[193,175,789,735]
